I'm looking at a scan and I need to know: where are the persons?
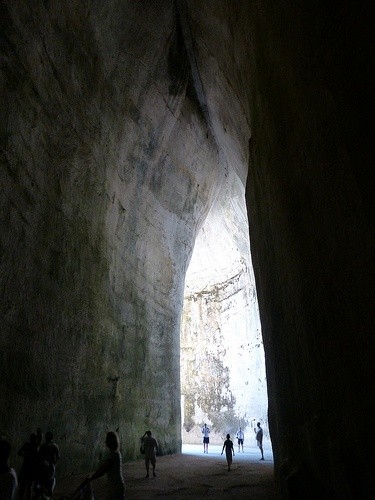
[84,432,125,500]
[0,439,18,500]
[254,422,264,460]
[17,428,59,500]
[221,434,235,469]
[141,431,158,475]
[237,427,244,453]
[202,424,210,454]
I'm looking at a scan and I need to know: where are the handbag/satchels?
[139,446,145,454]
